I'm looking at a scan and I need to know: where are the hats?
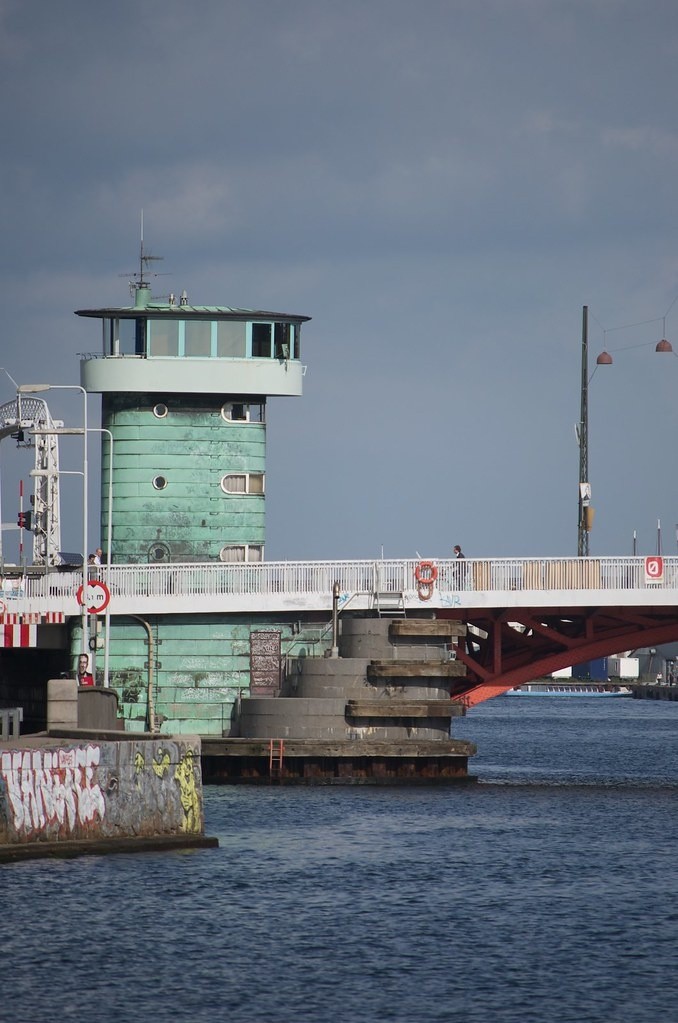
[89,554,96,558]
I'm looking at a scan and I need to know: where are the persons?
[453,545,467,590]
[94,549,103,564]
[79,654,92,677]
[87,554,96,564]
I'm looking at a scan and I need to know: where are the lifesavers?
[415,561,438,600]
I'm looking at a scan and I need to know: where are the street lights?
[29,428,114,688]
[30,469,87,671]
[17,383,89,671]
